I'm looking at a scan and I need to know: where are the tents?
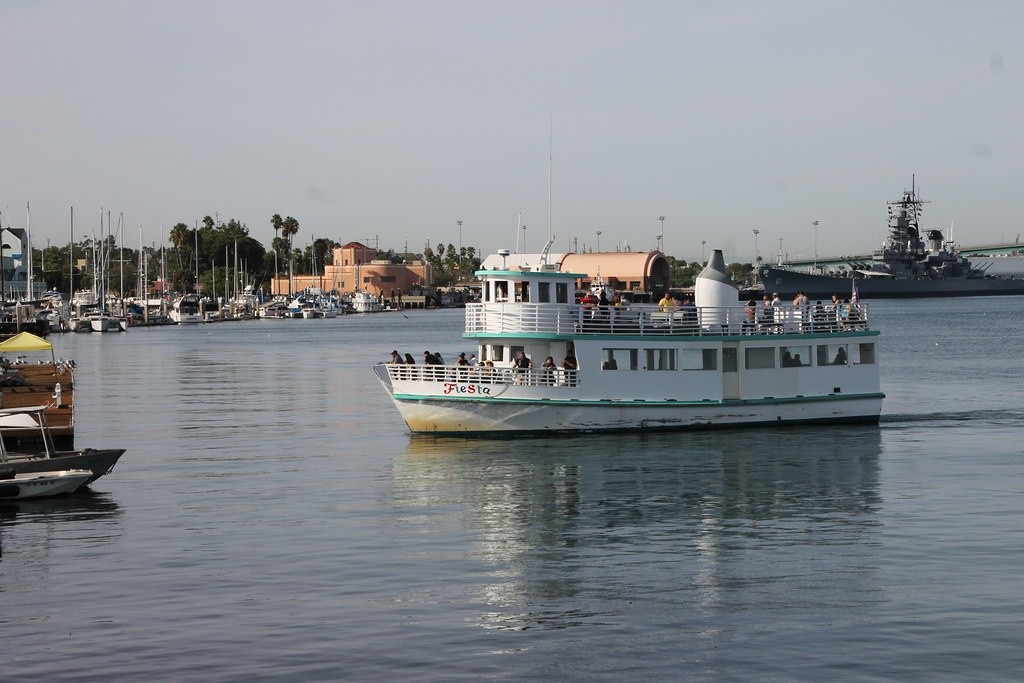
[0,332,57,381]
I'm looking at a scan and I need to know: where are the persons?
[450,353,496,383]
[603,359,617,369]
[386,350,406,380]
[834,347,846,364]
[541,357,557,386]
[581,290,632,324]
[658,294,697,324]
[424,351,445,381]
[564,350,577,387]
[474,288,482,301]
[784,352,800,366]
[758,293,781,327]
[512,352,532,385]
[742,300,756,335]
[793,290,861,332]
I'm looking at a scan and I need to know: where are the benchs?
[647,312,868,335]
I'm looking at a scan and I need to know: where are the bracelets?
[388,362,389,364]
[404,353,417,380]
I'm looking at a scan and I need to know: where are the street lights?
[457,220,463,265]
[596,230,602,253]
[522,224,527,253]
[812,220,819,276]
[656,235,662,251]
[701,240,705,266]
[659,215,666,253]
[753,229,759,285]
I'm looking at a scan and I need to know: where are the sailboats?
[0,200,386,342]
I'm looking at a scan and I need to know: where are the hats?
[772,293,778,295]
[390,350,397,354]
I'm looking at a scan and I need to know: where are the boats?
[754,171,1024,303]
[0,467,95,503]
[0,432,127,489]
[0,329,76,443]
[370,247,888,438]
